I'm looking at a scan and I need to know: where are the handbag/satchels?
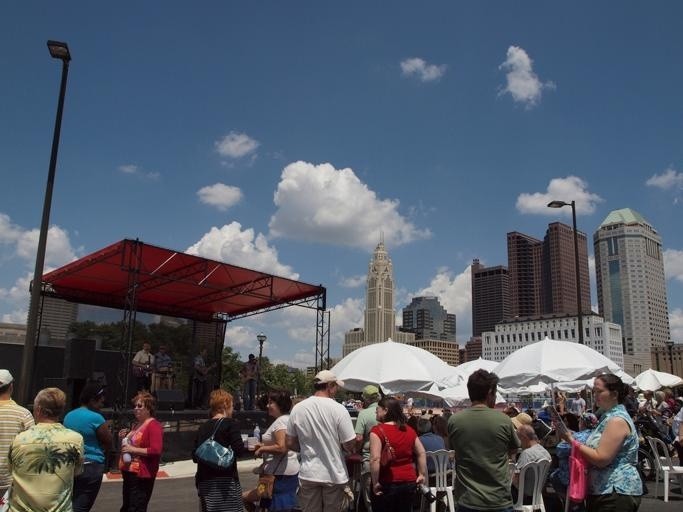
[256,474,275,500]
[378,425,396,467]
[567,441,586,503]
[193,417,235,472]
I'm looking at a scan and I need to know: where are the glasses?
[133,403,143,408]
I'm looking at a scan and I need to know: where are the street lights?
[253,332,269,406]
[12,37,74,408]
[550,198,593,347]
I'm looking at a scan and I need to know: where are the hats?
[416,419,431,433]
[313,369,344,388]
[510,412,533,429]
[0,368,16,388]
[362,385,379,400]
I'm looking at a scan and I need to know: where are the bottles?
[122,438,132,464]
[253,424,260,442]
[171,405,175,416]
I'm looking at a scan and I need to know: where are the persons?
[115,388,164,512]
[153,343,172,393]
[63,383,115,512]
[6,384,85,512]
[0,368,35,512]
[510,374,681,512]
[447,369,521,512]
[239,353,260,411]
[130,341,156,412]
[190,346,215,411]
[255,369,451,512]
[190,388,266,512]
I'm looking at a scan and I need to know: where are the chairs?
[645,436,682,502]
[419,451,455,511]
[511,458,551,512]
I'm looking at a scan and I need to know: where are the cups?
[241,434,249,443]
[247,437,257,451]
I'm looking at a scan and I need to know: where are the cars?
[345,402,359,413]
